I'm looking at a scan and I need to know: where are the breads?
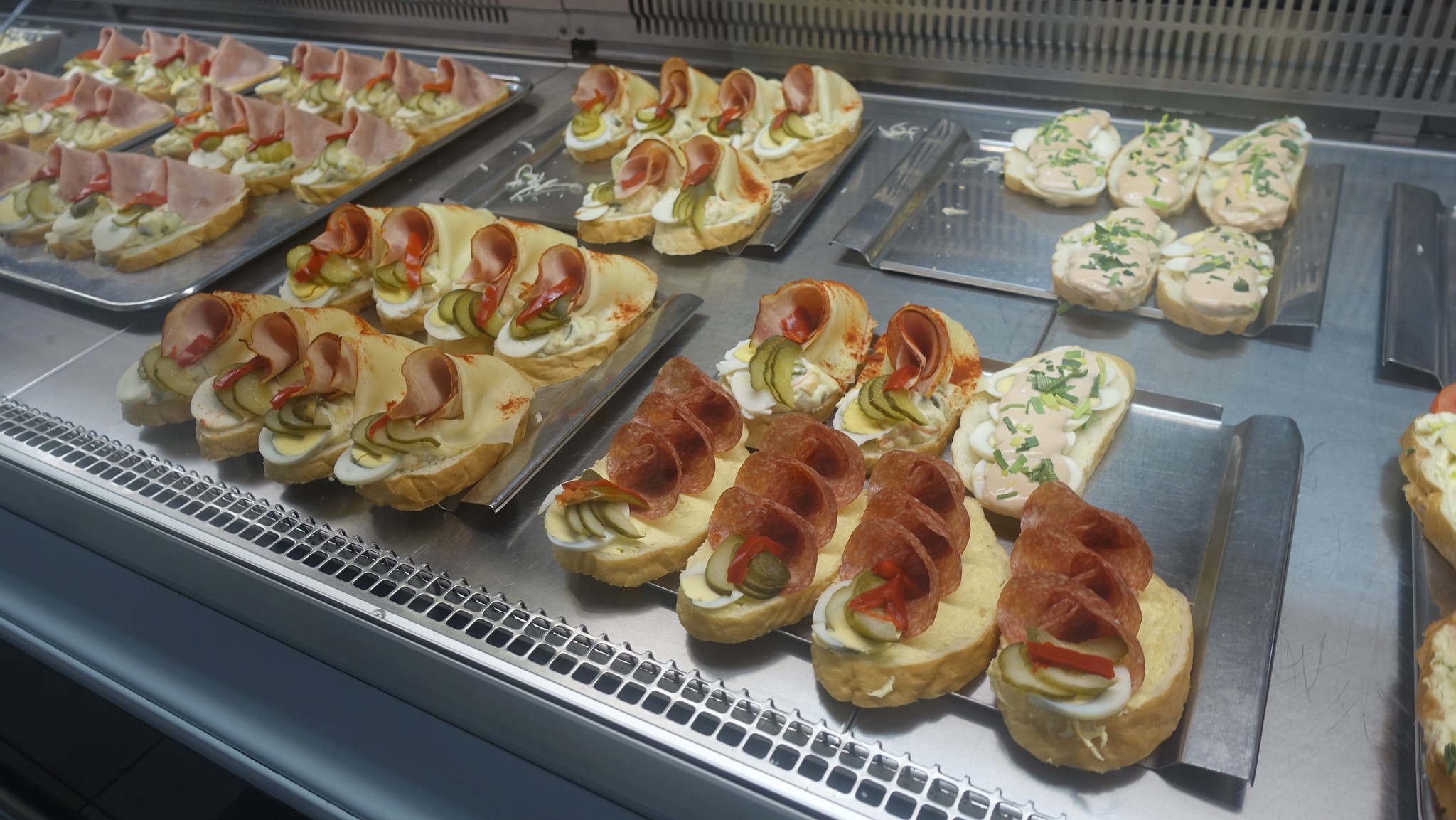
[0,24,1456,820]
